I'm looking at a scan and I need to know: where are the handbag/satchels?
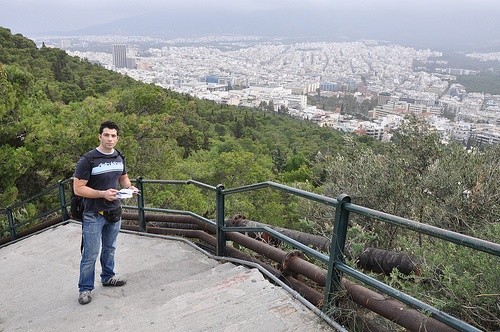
[103,207,122,223]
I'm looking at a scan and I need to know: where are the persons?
[73,121,138,305]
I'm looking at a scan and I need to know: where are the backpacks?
[70,149,125,223]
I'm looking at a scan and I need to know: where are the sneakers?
[79,290,92,305]
[102,275,127,287]
